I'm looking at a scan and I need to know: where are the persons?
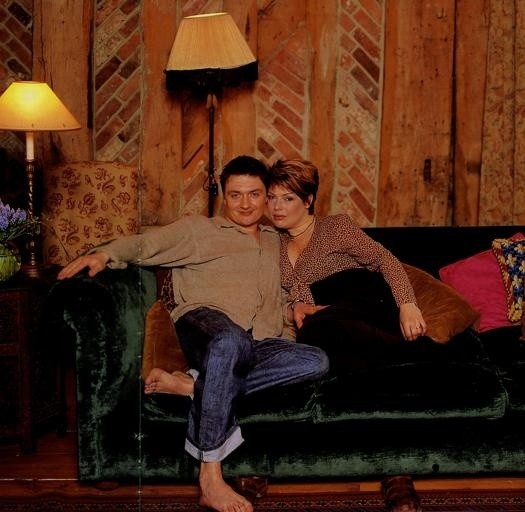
[56,155,333,512]
[265,156,501,382]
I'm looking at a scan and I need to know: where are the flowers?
[0,197,40,242]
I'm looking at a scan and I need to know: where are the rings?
[311,303,316,307]
[415,326,422,329]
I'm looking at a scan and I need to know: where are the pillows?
[38,159,140,267]
[400,232,525,342]
[140,299,192,383]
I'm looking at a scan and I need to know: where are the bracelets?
[286,298,307,310]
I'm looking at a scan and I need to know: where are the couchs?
[57,225,525,487]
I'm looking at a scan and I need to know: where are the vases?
[0,243,22,282]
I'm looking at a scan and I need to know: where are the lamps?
[162,14,259,219]
[0,81,82,279]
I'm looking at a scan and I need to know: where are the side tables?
[0,267,76,444]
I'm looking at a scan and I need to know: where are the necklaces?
[286,215,317,238]
